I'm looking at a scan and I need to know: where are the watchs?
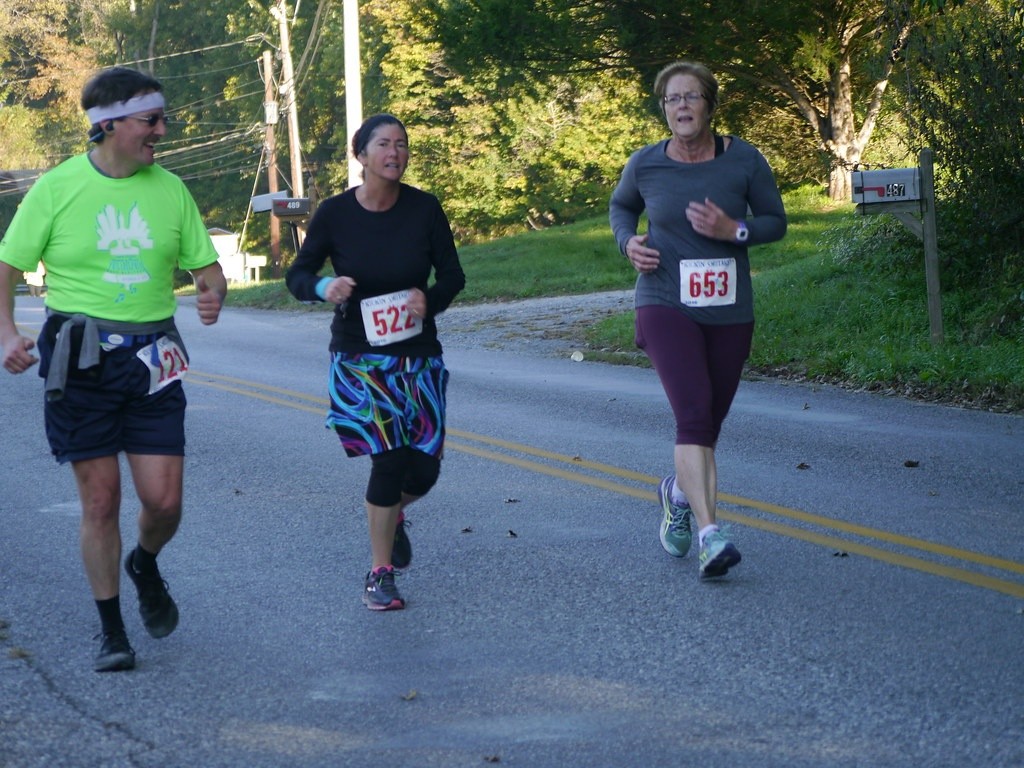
[734,219,749,247]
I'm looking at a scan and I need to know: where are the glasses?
[665,90,710,106]
[126,114,170,128]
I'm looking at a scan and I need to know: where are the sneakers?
[93,628,135,672]
[697,531,741,577]
[658,475,694,558]
[124,549,179,640]
[362,566,405,611]
[390,510,412,568]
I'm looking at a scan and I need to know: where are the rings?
[410,309,418,317]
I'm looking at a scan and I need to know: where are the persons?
[286,114,466,612]
[609,61,787,583]
[0,65,228,672]
[23,260,46,297]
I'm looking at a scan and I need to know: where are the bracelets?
[315,277,335,302]
